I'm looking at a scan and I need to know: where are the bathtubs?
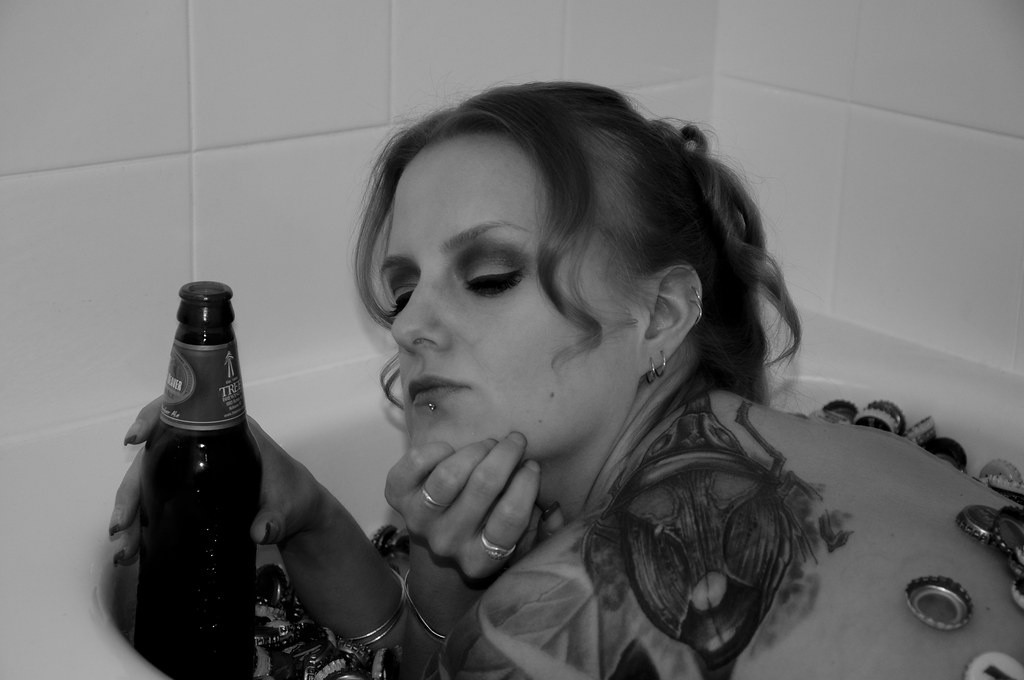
[3,303,1022,679]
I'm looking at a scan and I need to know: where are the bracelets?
[339,568,407,648]
[403,568,447,645]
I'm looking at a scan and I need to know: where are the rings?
[479,529,516,561]
[422,486,450,510]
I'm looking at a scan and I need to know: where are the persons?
[107,80,1024,680]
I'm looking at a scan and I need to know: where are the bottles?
[136,281,260,680]
